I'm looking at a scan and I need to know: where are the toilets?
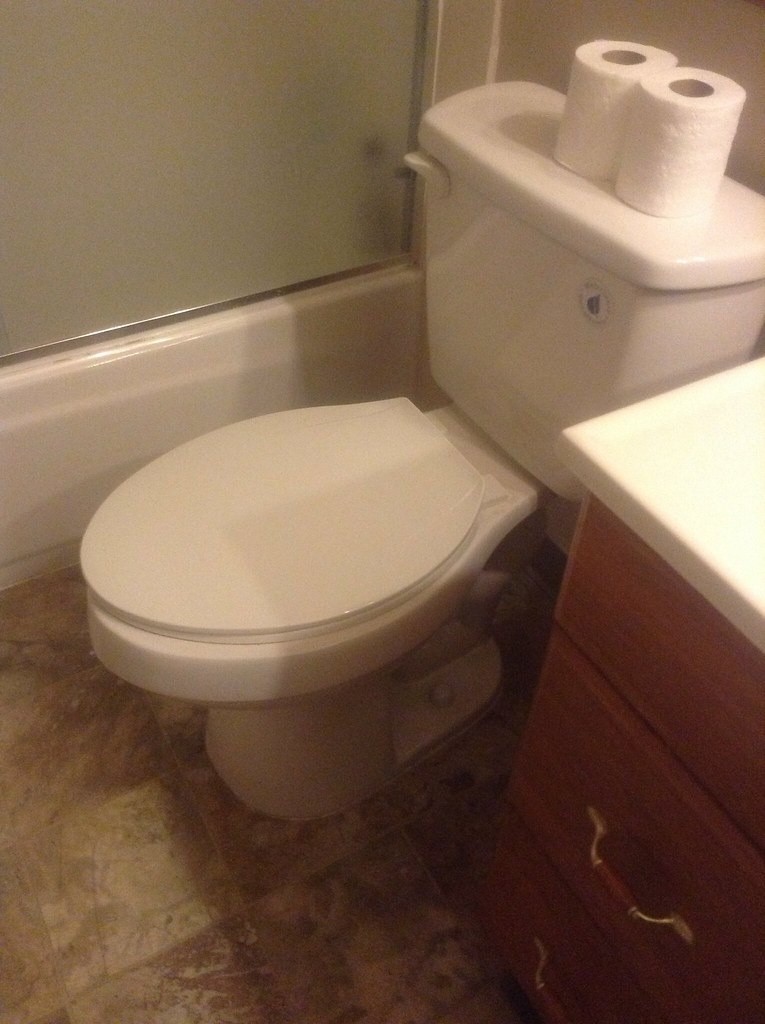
[80,81,765,815]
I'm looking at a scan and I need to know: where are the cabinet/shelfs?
[465,488,764,1024]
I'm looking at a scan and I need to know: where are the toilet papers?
[554,41,677,182]
[616,63,745,219]
